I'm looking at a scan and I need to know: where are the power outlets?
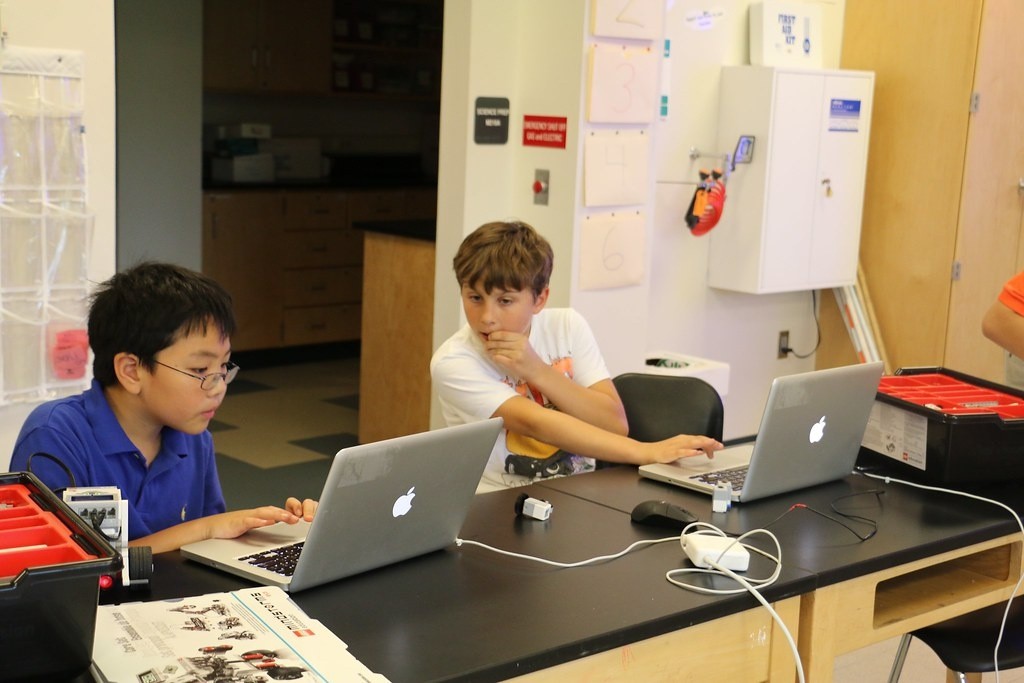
[777,330,790,359]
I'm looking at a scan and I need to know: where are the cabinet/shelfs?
[709,65,877,295]
[202,191,436,352]
[202,1,330,100]
[330,0,444,104]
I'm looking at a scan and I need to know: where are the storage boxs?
[0,471,124,683]
[260,136,325,180]
[856,364,1023,498]
[215,138,258,159]
[217,123,273,139]
[211,154,274,183]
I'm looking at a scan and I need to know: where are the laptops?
[179,417,504,593]
[638,360,884,503]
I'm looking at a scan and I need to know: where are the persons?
[431,221,724,494]
[981,271,1024,362]
[8,262,320,554]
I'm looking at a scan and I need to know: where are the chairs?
[594,373,725,472]
[888,595,1024,683]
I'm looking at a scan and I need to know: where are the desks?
[139,428,1022,683]
[353,217,436,445]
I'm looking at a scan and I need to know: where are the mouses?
[631,499,699,530]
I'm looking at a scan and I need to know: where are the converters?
[682,535,750,572]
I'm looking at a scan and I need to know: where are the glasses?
[151,359,240,390]
